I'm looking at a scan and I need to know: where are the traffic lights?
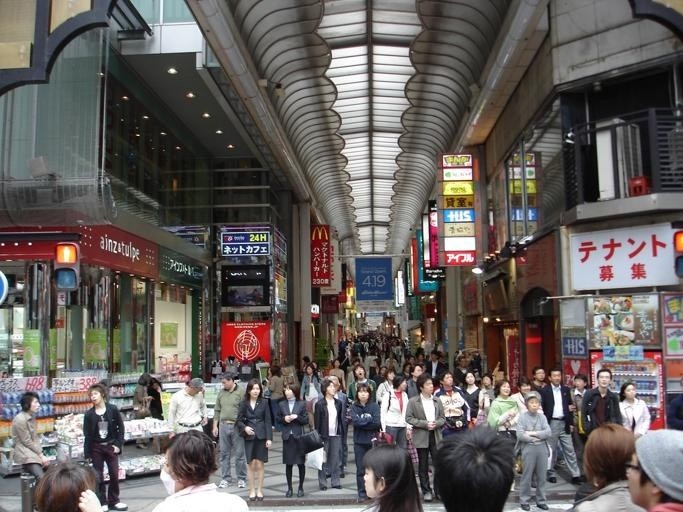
[672,227,683,278]
[51,239,80,293]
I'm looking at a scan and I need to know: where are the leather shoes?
[549,477,557,482]
[250,497,263,501]
[286,489,292,497]
[537,504,549,510]
[572,477,581,484]
[521,504,530,511]
[297,489,304,497]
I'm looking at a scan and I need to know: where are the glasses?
[624,457,638,474]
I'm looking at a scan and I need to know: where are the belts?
[180,421,200,427]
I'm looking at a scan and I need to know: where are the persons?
[11,330,683,512]
[227,288,261,306]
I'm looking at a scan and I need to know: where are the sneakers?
[109,503,128,510]
[101,505,109,512]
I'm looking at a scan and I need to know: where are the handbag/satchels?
[499,431,517,447]
[305,447,324,470]
[234,417,246,437]
[446,415,465,431]
[294,430,324,456]
[137,408,151,418]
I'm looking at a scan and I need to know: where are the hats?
[635,430,682,500]
[189,378,207,392]
